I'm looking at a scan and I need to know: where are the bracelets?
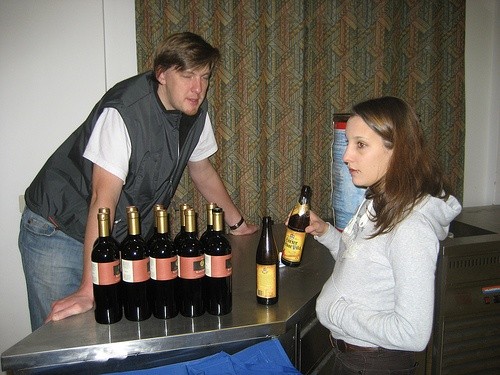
[229,216,245,230]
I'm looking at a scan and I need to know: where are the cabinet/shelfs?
[0,202,500,375]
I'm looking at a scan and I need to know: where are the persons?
[284,97,462,375]
[18,32,260,334]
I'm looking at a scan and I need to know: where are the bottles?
[174,204,190,247]
[91,207,123,323]
[281,185,311,267]
[204,207,232,315]
[255,216,279,305]
[120,206,148,320]
[177,208,204,317]
[148,203,163,247]
[200,201,216,243]
[148,210,178,318]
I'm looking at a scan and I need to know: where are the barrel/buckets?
[334,112,369,233]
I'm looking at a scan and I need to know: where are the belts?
[327,334,377,354]
